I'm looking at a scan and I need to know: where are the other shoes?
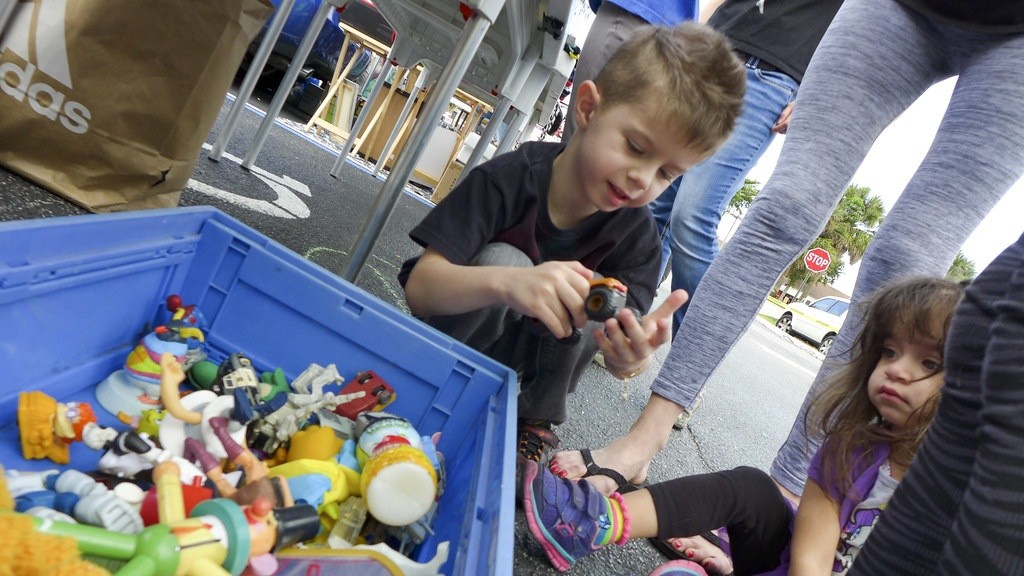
[593,347,607,370]
[673,397,703,429]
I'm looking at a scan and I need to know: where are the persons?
[401,19,748,507]
[842,238,1024,575]
[551,0,1024,576]
[524,273,973,575]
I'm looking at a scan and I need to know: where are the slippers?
[551,448,650,494]
[649,529,733,574]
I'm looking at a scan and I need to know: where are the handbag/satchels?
[0,0,276,217]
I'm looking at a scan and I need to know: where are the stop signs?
[803,248,831,273]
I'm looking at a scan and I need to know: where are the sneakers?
[649,558,708,576]
[516,420,561,505]
[522,460,607,572]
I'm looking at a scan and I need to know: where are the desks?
[206,0,584,289]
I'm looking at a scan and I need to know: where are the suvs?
[775,295,851,356]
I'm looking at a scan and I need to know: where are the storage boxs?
[0,201,520,576]
